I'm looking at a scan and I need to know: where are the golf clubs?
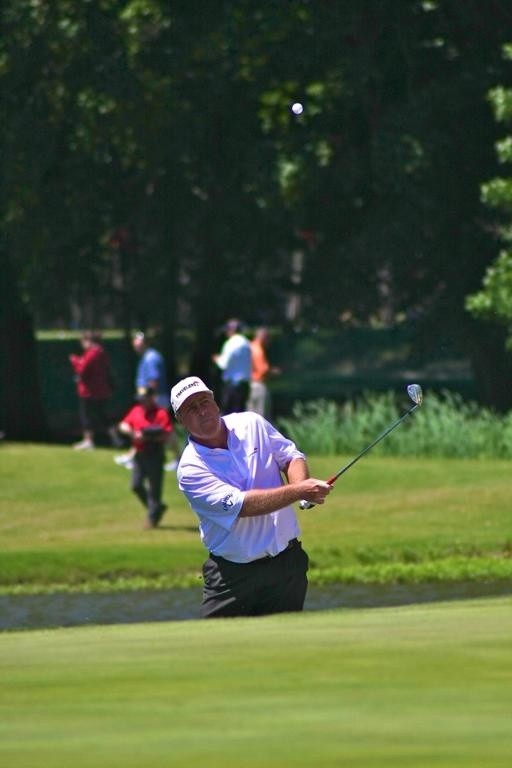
[301,382,423,511]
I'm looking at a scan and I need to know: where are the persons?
[119,384,174,527]
[69,330,112,450]
[212,320,252,414]
[114,328,181,470]
[244,327,281,416]
[170,376,334,618]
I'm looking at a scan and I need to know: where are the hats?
[170,377,213,414]
